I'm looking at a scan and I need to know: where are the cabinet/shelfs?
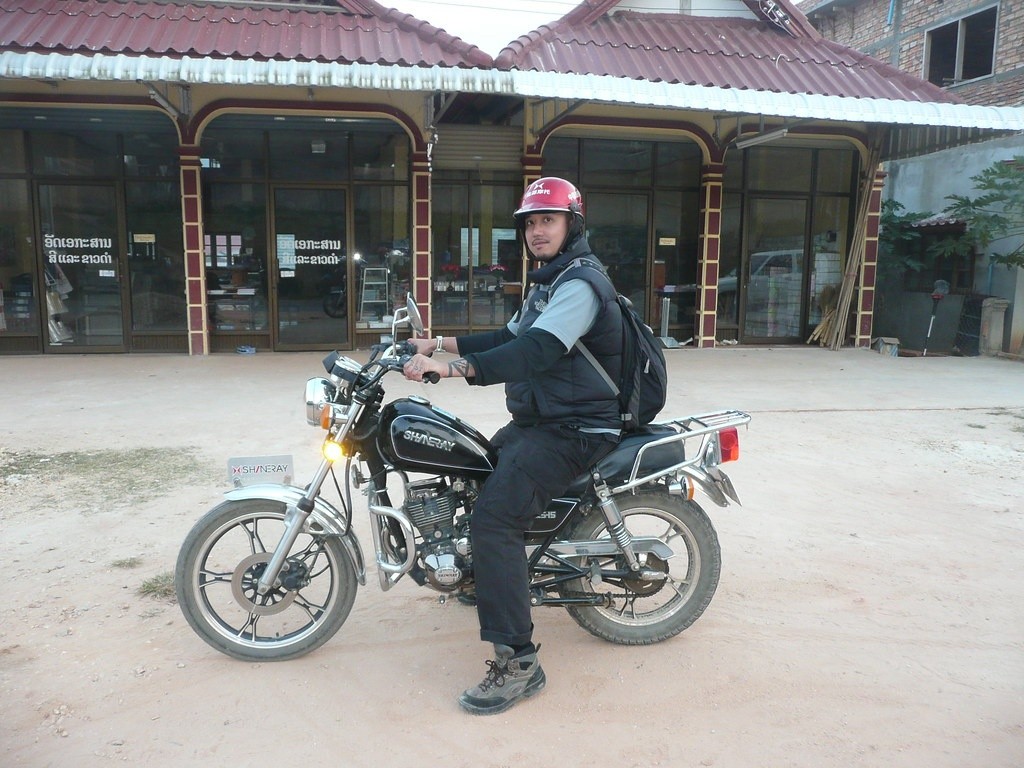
[359,267,389,322]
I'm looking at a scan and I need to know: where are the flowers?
[490,264,507,279]
[441,264,459,281]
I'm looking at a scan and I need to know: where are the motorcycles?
[174,294,752,662]
[324,252,404,319]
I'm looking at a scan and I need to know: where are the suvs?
[667,249,824,330]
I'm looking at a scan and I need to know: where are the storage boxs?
[2,272,36,332]
[871,336,899,357]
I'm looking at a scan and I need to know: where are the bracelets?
[435,336,445,352]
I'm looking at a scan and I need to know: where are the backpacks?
[547,257,667,430]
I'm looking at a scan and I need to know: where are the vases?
[495,280,504,290]
[446,282,455,292]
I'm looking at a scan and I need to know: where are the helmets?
[513,177,586,223]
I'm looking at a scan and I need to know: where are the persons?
[403,177,625,716]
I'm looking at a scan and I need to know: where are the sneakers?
[457,642,546,715]
[457,583,545,606]
